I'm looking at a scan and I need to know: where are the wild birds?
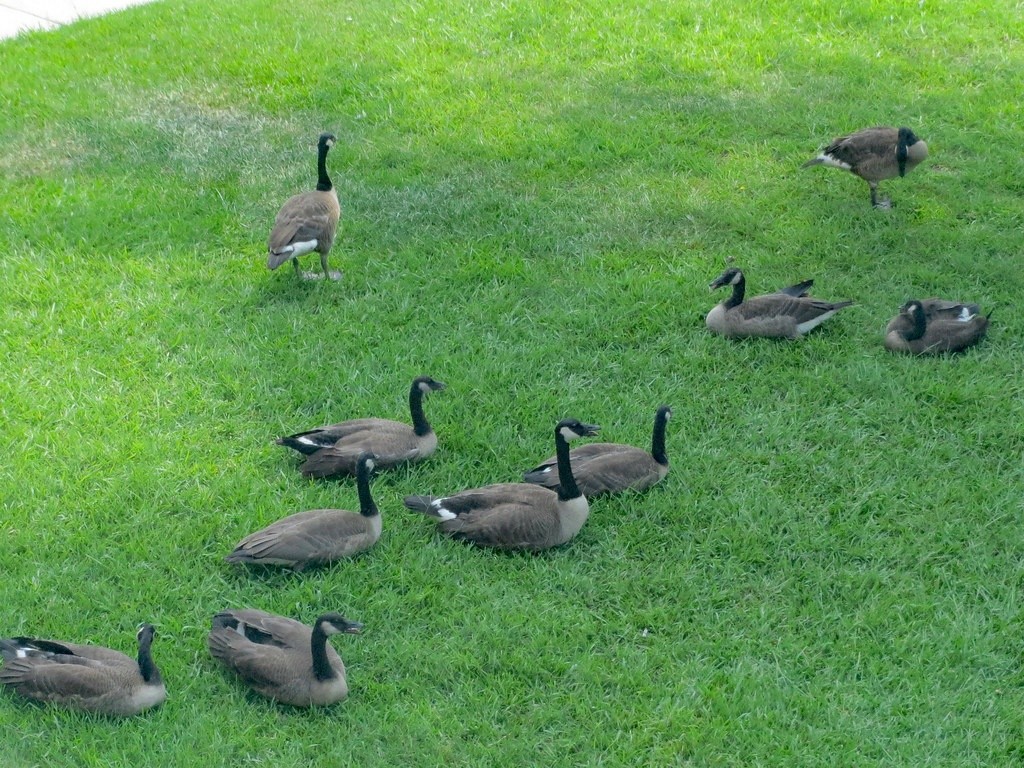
[277,376,448,480]
[523,405,673,509]
[403,417,602,552]
[226,449,383,573]
[0,621,167,721]
[801,126,928,209]
[704,266,855,342]
[209,608,364,708]
[268,133,342,282]
[885,297,988,357]
